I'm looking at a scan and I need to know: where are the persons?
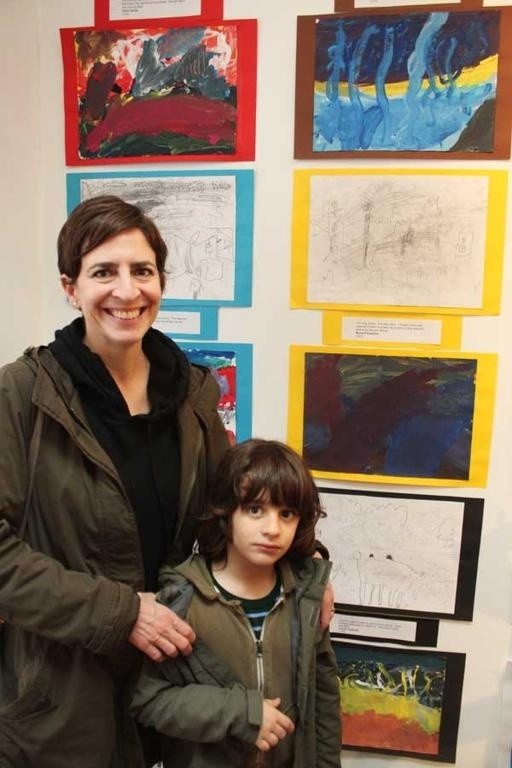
[0,195,232,768]
[119,438,341,767]
